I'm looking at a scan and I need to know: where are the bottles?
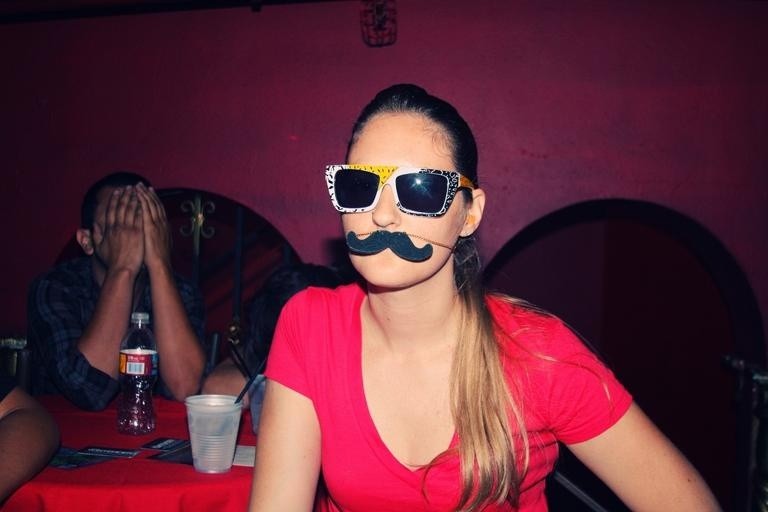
[116,312,159,436]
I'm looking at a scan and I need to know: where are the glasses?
[323,162,475,219]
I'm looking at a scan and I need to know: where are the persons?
[1,366,60,506]
[247,84,724,511]
[199,263,352,409]
[29,170,215,412]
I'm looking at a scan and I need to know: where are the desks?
[0,393,260,512]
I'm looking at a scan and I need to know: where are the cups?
[183,394,244,474]
